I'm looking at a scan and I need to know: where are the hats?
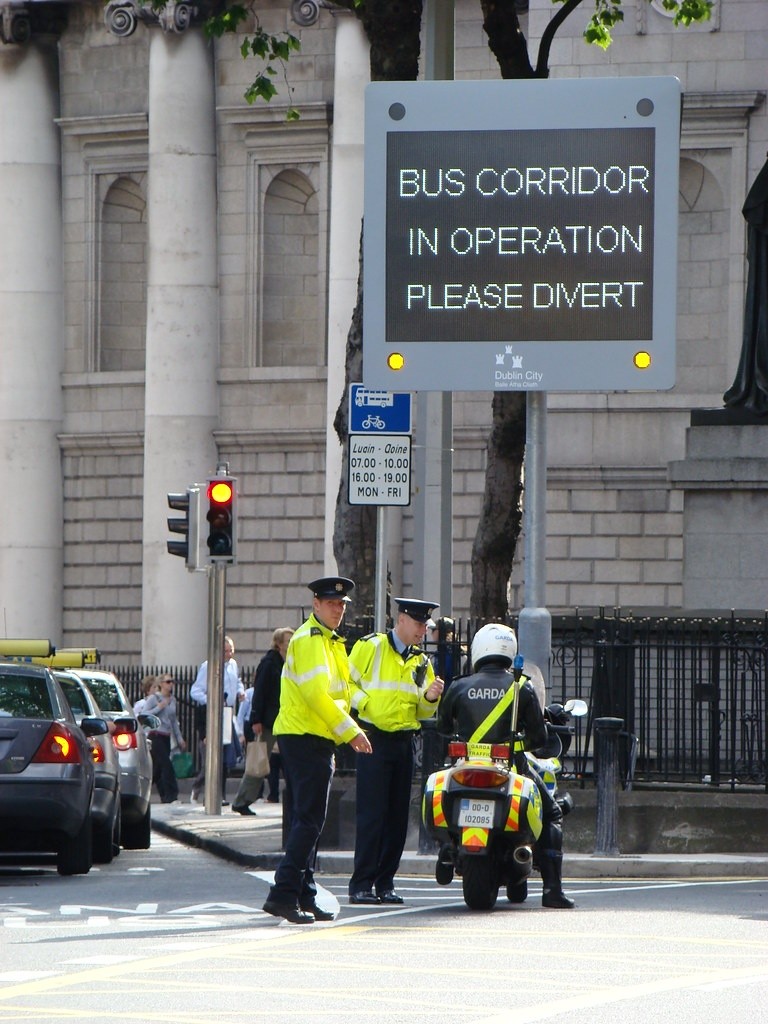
[394,597,440,626]
[307,577,355,601]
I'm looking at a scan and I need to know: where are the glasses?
[162,680,173,683]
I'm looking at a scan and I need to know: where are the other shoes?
[436,846,458,885]
[542,889,575,907]
[232,805,256,816]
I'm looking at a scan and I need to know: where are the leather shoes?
[301,905,334,920]
[375,888,403,903]
[349,890,383,903]
[263,900,315,923]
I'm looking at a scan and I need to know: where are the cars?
[1,639,161,876]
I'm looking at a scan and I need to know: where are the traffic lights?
[167,488,199,567]
[204,475,237,560]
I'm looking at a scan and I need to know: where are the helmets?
[471,624,517,672]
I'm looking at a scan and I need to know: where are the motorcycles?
[420,654,588,910]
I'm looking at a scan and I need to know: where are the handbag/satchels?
[172,752,195,778]
[245,732,271,776]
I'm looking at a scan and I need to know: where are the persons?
[128,626,303,818]
[264,577,375,924]
[409,616,465,805]
[435,624,576,908]
[347,597,445,905]
